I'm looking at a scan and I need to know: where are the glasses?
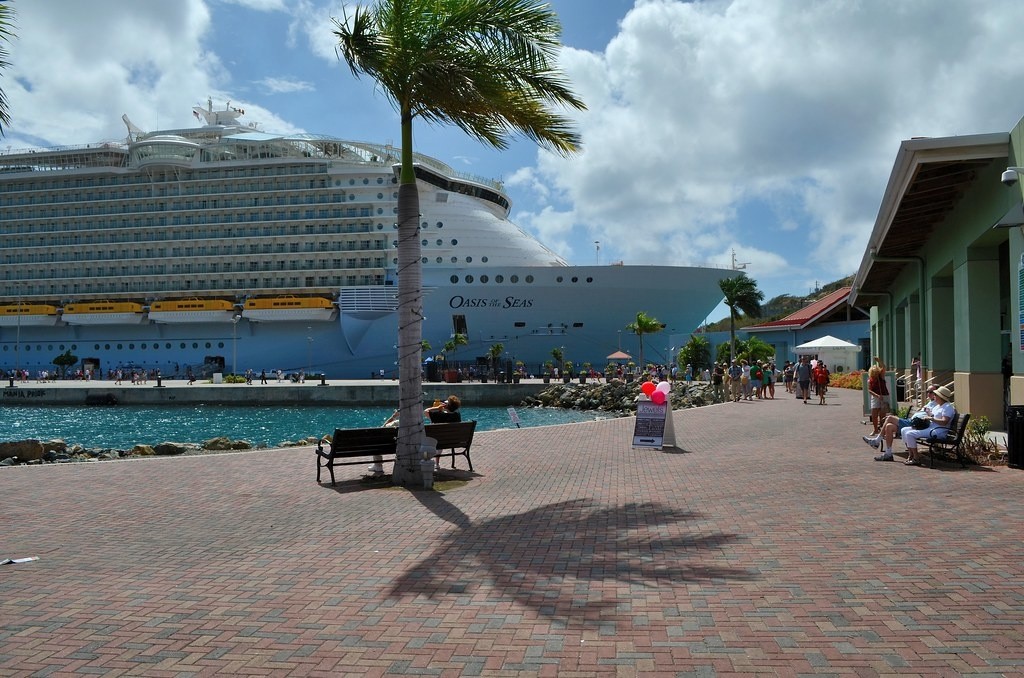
[926,389,933,393]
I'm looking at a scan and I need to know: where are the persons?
[8,368,305,387]
[862,383,938,461]
[911,357,922,388]
[368,410,398,472]
[459,358,830,405]
[901,386,955,467]
[423,396,461,469]
[868,356,890,436]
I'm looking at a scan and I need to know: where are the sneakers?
[873,453,894,461]
[862,435,880,450]
[903,456,921,466]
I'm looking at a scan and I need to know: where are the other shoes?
[368,462,383,471]
[870,431,878,435]
[435,462,440,468]
[733,396,740,402]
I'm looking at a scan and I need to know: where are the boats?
[0,301,58,326]
[147,297,236,323]
[241,297,339,322]
[61,301,144,325]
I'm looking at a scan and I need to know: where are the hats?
[933,386,951,402]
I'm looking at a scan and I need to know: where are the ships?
[0,96,749,381]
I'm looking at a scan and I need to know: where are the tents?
[794,334,862,374]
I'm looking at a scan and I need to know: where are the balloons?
[652,390,665,404]
[656,381,670,395]
[642,382,655,396]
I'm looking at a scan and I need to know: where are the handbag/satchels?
[911,416,929,429]
[880,401,890,413]
[741,376,748,385]
[756,369,762,378]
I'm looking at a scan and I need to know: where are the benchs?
[916,412,972,469]
[315,419,477,487]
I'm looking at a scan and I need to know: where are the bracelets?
[392,415,396,421]
[931,417,934,421]
